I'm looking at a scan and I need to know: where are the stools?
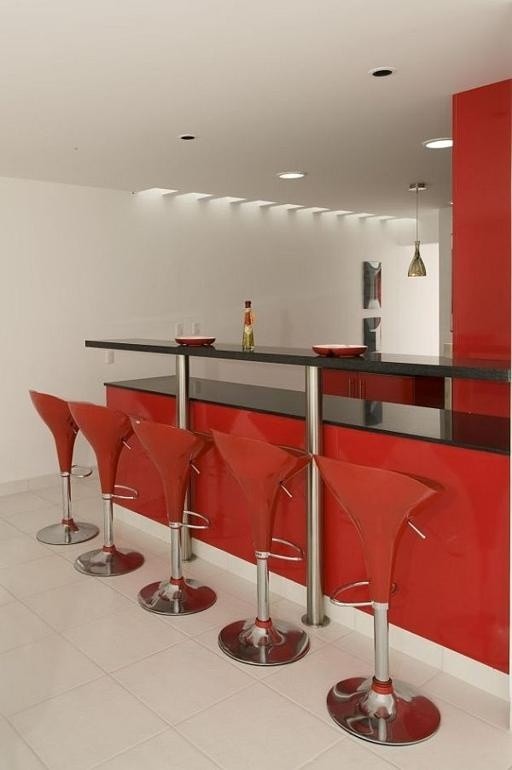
[209,425,311,668]
[67,399,145,576]
[129,414,218,616]
[29,388,101,545]
[311,452,441,746]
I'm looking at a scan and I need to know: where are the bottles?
[241,300,255,349]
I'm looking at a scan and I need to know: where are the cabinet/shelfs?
[320,367,444,408]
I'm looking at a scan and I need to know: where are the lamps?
[405,181,427,277]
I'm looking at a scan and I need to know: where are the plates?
[312,344,368,358]
[175,337,216,345]
